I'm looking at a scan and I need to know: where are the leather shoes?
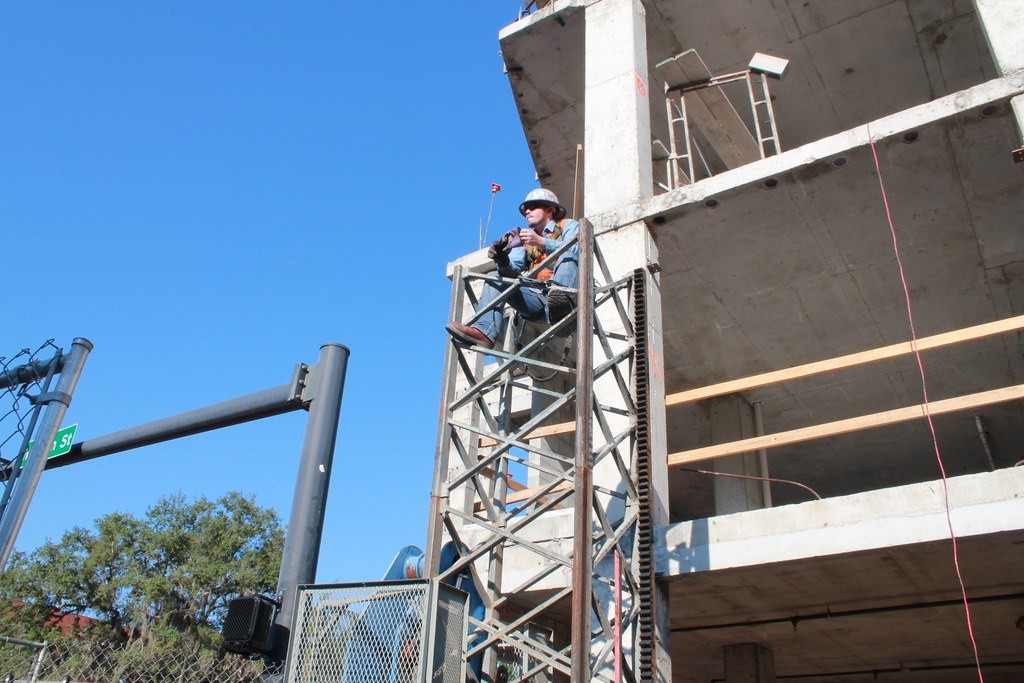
[549,290,575,338]
[445,321,495,350]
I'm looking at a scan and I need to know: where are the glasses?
[524,203,549,210]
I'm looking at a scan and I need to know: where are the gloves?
[488,241,511,267]
[501,226,522,251]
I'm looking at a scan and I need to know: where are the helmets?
[519,189,566,221]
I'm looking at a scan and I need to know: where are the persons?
[445,189,580,350]
